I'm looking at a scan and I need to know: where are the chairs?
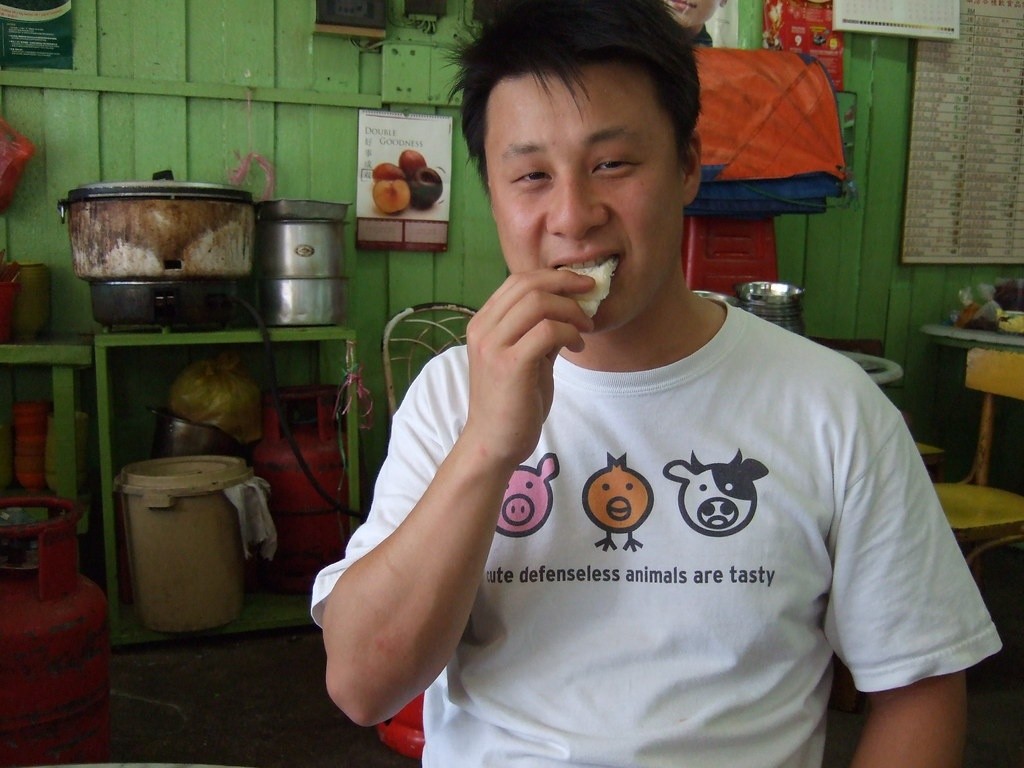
[934,345,1024,590]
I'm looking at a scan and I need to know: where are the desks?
[923,321,1024,353]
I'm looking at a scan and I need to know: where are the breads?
[555,256,618,318]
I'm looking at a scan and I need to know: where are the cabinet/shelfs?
[93,324,364,647]
[0,330,99,563]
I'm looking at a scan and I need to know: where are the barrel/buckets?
[118,457,258,631]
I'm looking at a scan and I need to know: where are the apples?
[372,151,444,217]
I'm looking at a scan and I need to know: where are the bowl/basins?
[691,281,807,336]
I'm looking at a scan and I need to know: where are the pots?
[258,219,351,275]
[60,167,256,277]
[146,406,238,458]
[259,277,349,327]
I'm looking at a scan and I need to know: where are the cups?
[0,283,17,345]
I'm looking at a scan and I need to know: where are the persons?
[664,0,728,48]
[311,0,1002,768]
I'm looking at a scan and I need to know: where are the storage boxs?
[763,0,844,91]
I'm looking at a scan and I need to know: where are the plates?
[260,200,353,220]
